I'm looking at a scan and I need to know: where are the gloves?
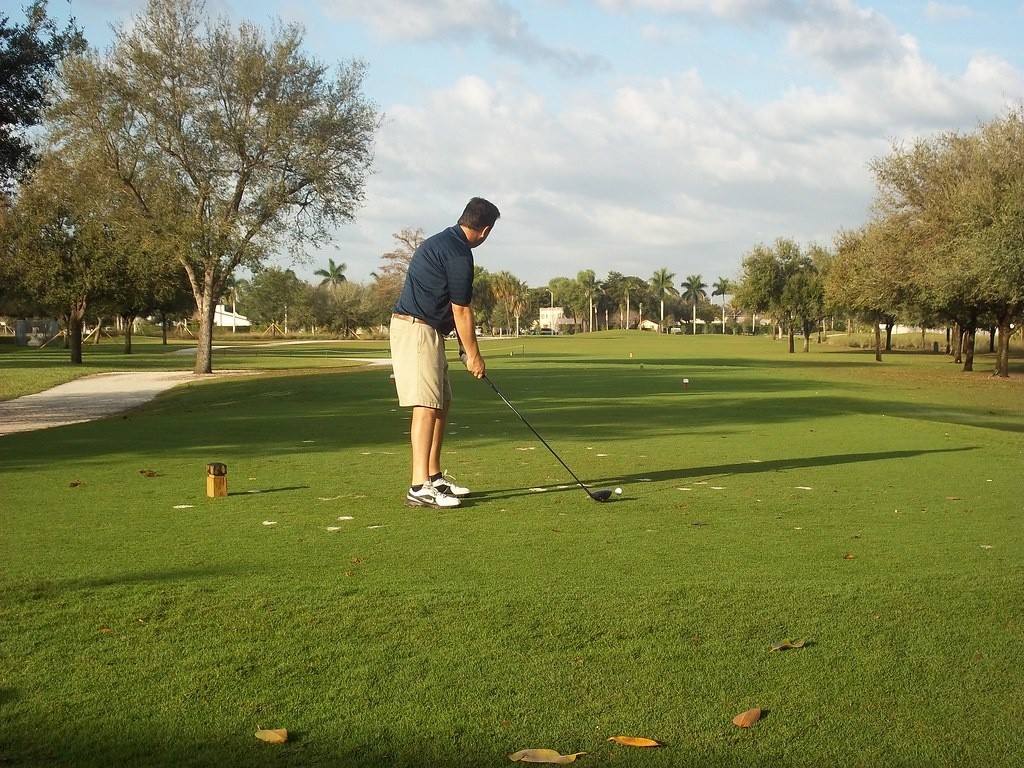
[460,345,468,365]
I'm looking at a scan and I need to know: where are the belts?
[393,314,426,324]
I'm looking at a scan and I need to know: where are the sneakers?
[407,477,471,509]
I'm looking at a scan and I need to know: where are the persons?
[387,195,503,509]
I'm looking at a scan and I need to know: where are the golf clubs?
[459,350,612,502]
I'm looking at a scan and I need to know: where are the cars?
[530,327,558,335]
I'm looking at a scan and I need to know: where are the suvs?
[450,327,482,338]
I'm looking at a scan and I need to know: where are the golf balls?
[615,488,623,495]
